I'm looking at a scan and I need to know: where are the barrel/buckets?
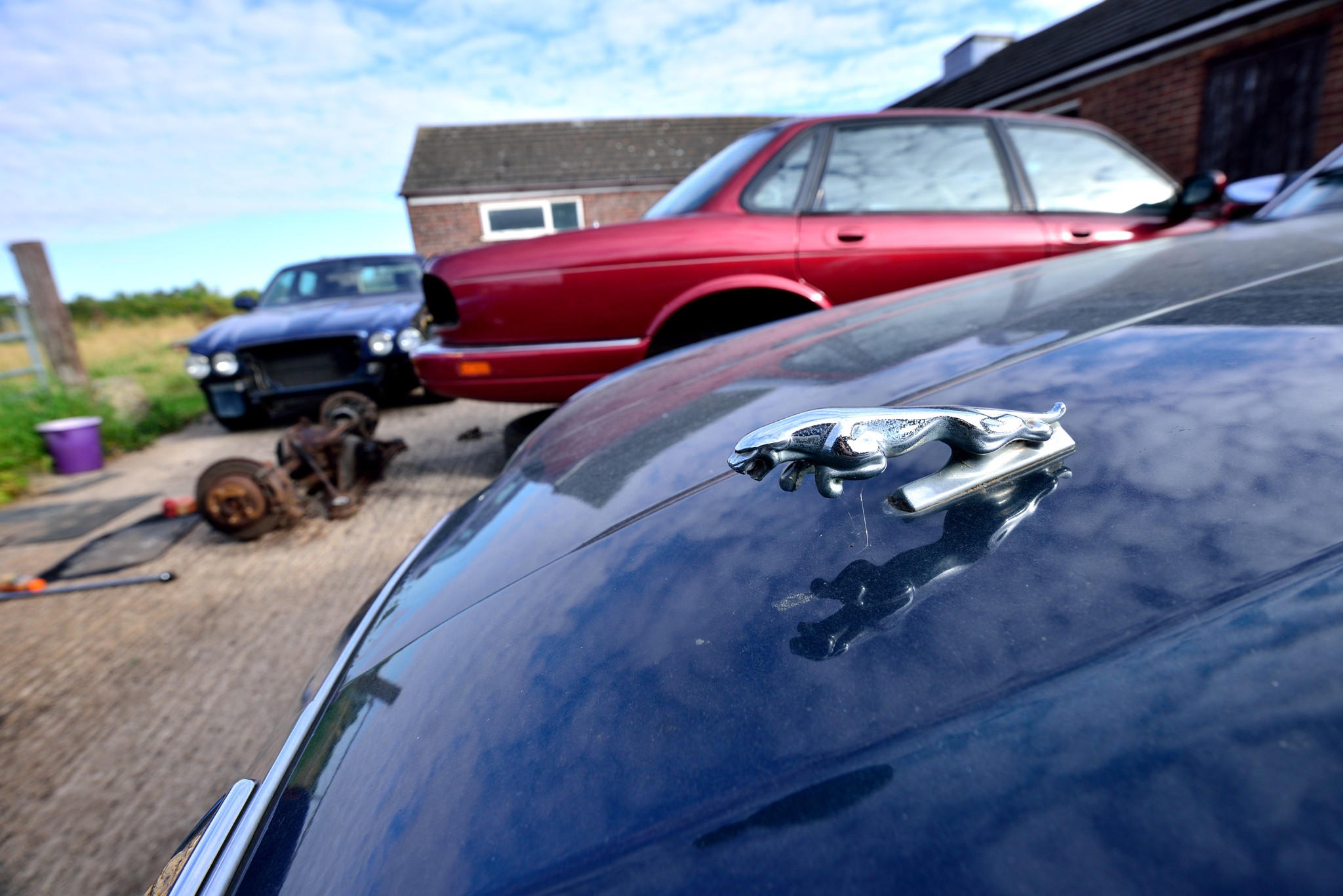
[33,416,105,474]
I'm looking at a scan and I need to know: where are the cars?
[182,253,455,432]
[408,104,1251,404]
[141,139,1343,896]
